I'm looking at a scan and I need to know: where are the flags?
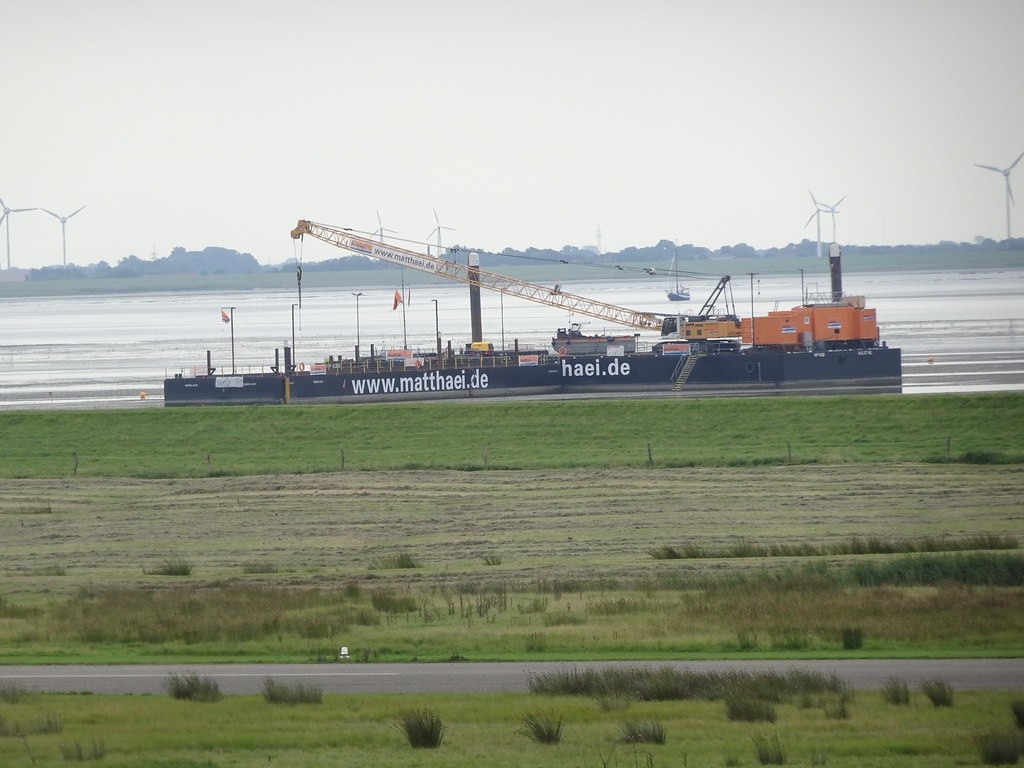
[392,290,402,310]
[221,310,230,323]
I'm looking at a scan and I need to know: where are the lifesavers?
[559,347,568,356]
[298,362,305,372]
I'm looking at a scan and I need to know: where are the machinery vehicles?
[288,213,743,353]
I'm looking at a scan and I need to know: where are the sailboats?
[664,243,692,302]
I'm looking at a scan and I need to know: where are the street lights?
[499,286,506,351]
[430,300,439,345]
[229,307,237,374]
[291,303,298,371]
[352,292,363,351]
[796,268,805,307]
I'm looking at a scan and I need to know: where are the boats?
[160,241,905,404]
[551,322,642,355]
